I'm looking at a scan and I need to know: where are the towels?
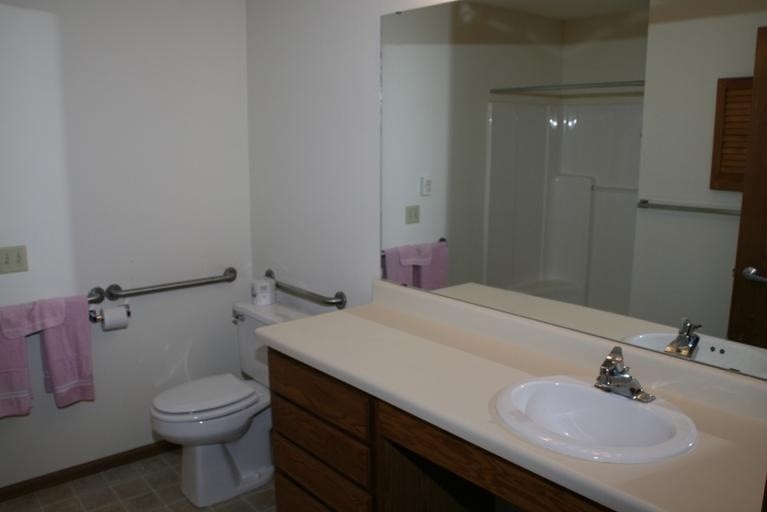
[382,242,447,295]
[0,294,95,420]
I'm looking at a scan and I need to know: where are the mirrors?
[379,0,767,385]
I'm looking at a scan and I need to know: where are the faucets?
[664,318,702,358]
[594,346,655,404]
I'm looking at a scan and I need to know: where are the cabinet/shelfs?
[267,349,377,511]
[372,394,594,510]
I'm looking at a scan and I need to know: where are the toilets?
[151,302,316,507]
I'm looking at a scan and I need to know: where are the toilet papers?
[100,306,128,330]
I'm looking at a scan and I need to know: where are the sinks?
[495,376,697,463]
[623,331,767,380]
